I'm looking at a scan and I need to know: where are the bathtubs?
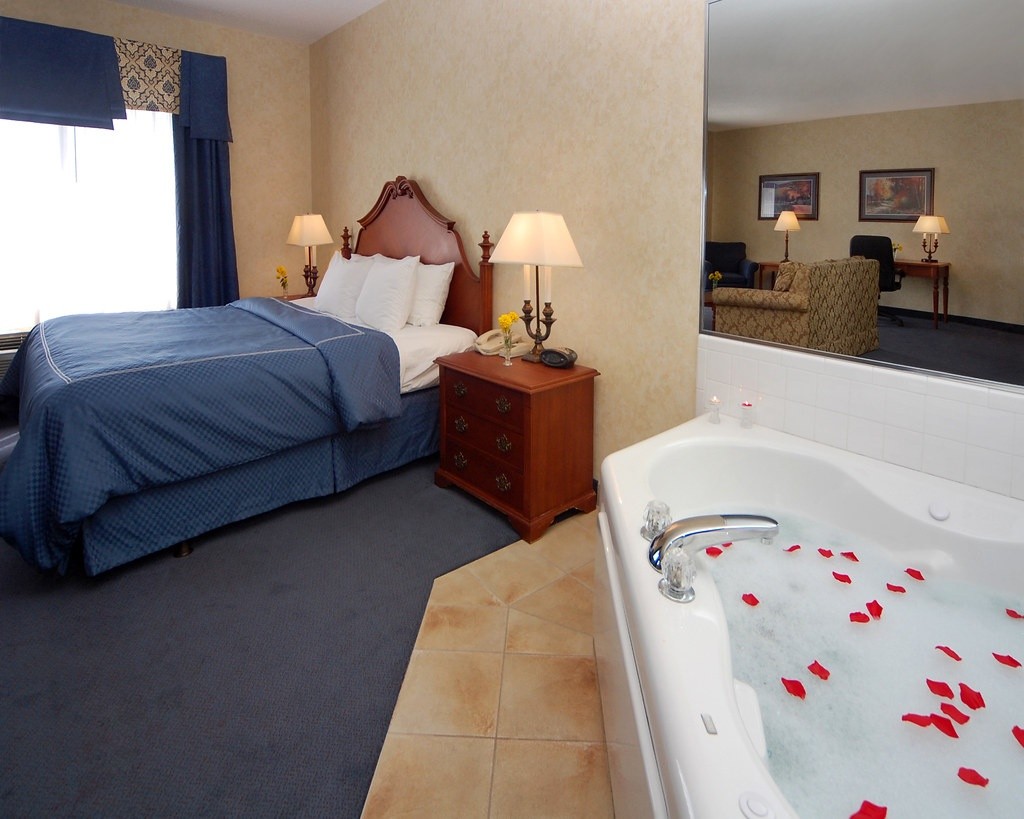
[651,439,1024,819]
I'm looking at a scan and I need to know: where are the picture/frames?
[858,169,935,223]
[757,172,819,221]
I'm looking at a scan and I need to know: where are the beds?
[0,176,495,580]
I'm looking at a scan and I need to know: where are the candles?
[707,395,721,425]
[740,400,753,429]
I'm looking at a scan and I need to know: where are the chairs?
[849,233,907,327]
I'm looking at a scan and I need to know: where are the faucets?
[648,513,780,575]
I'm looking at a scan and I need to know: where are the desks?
[758,261,779,290]
[893,258,950,328]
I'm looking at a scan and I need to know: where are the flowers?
[497,311,519,333]
[708,271,723,281]
[275,264,288,287]
[892,242,905,253]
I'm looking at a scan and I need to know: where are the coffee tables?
[704,290,713,307]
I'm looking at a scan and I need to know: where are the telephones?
[475,328,532,357]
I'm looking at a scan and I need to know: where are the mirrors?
[697,0,1024,394]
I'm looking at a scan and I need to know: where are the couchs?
[712,257,880,356]
[703,240,759,292]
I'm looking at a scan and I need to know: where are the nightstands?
[432,350,601,545]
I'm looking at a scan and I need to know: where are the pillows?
[355,255,421,337]
[312,249,374,320]
[406,261,456,327]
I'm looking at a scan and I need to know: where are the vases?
[283,285,289,299]
[893,248,897,262]
[713,279,718,290]
[502,334,513,367]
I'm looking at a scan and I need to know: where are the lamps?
[773,210,801,263]
[912,214,951,263]
[488,208,584,364]
[284,211,333,299]
